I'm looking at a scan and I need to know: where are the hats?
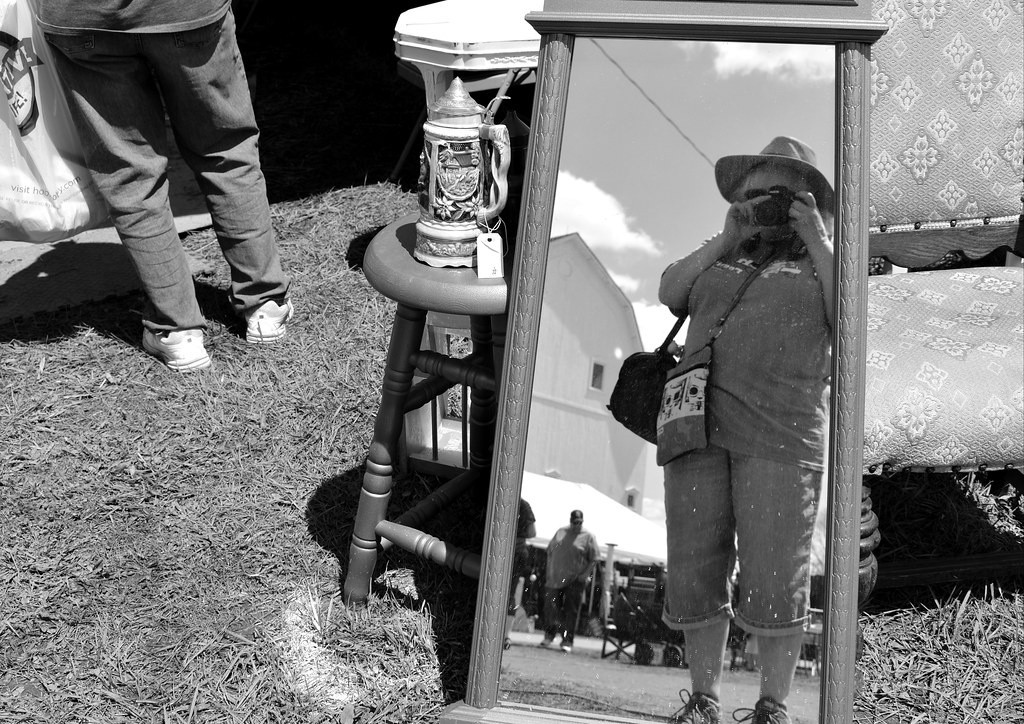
[715,136,834,217]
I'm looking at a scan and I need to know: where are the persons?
[35,0,293,375]
[502,498,536,649]
[538,510,599,652]
[658,136,834,724]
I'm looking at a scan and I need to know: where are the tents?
[521,471,667,566]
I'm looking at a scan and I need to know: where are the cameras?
[756,186,805,227]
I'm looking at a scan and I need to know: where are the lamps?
[412,76,511,268]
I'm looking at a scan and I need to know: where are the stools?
[342,211,512,606]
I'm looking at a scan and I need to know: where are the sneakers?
[245,298,294,344]
[142,327,212,373]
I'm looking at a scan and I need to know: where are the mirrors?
[441,0,892,724]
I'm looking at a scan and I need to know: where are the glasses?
[571,521,582,525]
[744,186,788,201]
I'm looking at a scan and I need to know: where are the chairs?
[856,0,1024,609]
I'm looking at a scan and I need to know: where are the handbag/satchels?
[656,344,712,466]
[0,0,110,243]
[606,347,677,445]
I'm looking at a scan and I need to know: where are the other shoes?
[540,639,551,646]
[504,638,512,650]
[733,696,792,724]
[561,645,571,651]
[668,689,722,724]
